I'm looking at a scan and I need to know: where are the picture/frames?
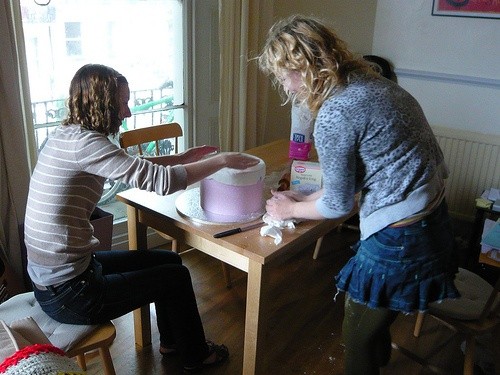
[431,0,500,20]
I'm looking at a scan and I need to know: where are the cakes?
[199,151,266,216]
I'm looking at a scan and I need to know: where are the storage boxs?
[290,160,322,196]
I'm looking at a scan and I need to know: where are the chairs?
[119,123,233,289]
[413,268,500,375]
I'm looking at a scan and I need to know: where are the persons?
[258,14,462,375]
[23,64,260,375]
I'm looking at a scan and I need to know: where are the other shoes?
[158,338,231,375]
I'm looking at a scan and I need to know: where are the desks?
[115,138,360,375]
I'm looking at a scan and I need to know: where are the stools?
[67,319,116,375]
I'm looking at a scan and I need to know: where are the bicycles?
[95,80,176,205]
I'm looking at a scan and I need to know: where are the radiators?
[428,122,500,219]
